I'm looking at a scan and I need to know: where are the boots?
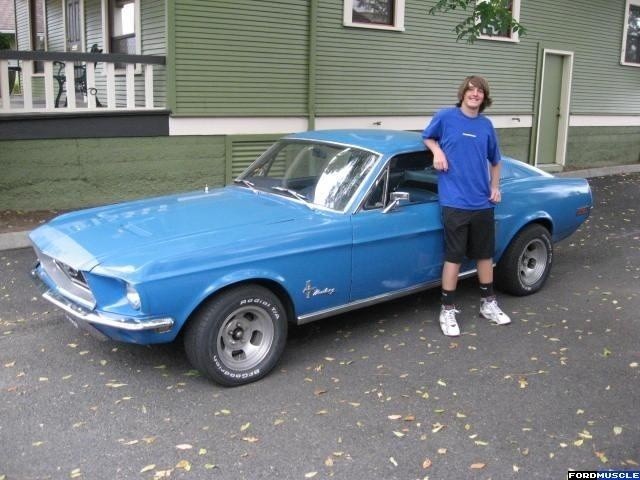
[480,297,511,324]
[438,305,460,337]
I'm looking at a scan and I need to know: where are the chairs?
[52,43,104,108]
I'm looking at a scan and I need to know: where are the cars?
[27,129,593,387]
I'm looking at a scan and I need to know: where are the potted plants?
[1,32,22,115]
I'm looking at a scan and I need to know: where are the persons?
[421,74,511,338]
